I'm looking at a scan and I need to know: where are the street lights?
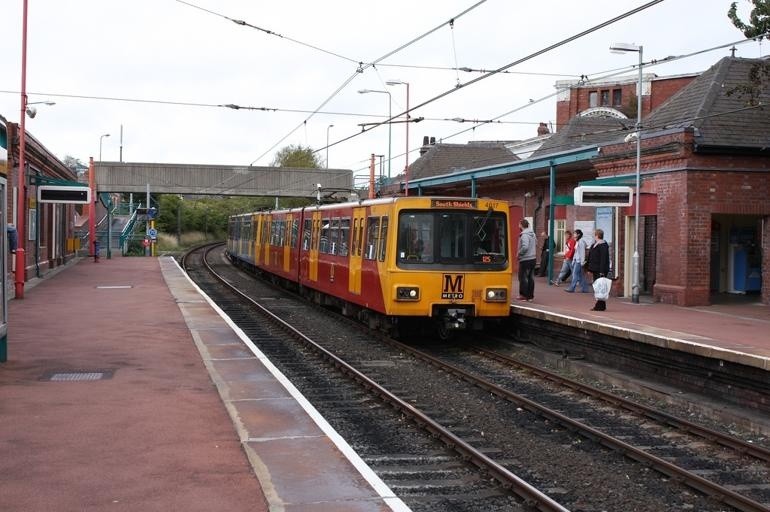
[608,41,645,305]
[326,124,335,169]
[358,89,392,185]
[384,80,410,198]
[100,133,110,161]
[15,96,56,300]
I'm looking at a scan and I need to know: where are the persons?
[564,230,588,293]
[514,220,537,302]
[402,227,425,259]
[587,228,609,311]
[550,230,576,286]
[536,232,556,277]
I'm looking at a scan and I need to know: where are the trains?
[224,194,513,347]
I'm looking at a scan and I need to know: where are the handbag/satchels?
[592,278,612,300]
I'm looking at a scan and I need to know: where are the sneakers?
[515,296,533,302]
[565,288,574,292]
[550,280,560,287]
